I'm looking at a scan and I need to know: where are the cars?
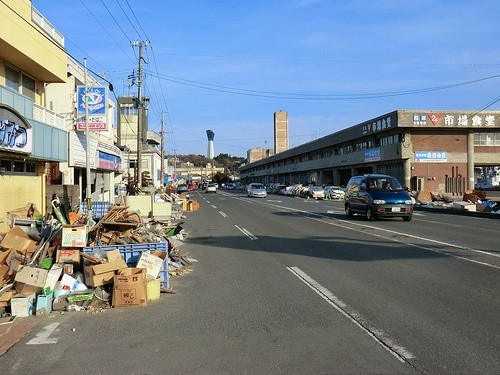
[203,182,219,193]
[177,183,197,192]
[247,183,267,198]
[268,182,345,199]
[226,182,236,191]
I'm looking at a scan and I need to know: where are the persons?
[384,182,393,190]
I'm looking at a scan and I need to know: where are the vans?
[344,174,413,221]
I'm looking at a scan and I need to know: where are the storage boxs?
[463,189,492,212]
[0,218,169,318]
